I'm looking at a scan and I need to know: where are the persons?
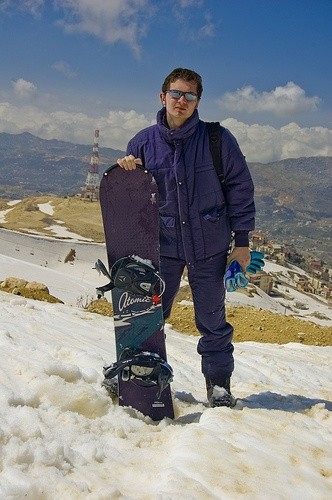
[117,68,255,407]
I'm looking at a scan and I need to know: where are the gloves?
[230,251,265,288]
[224,267,236,291]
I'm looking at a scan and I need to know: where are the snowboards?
[98,164,175,421]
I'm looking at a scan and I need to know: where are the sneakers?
[207,378,236,408]
[101,377,118,399]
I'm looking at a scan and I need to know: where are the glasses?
[166,89,199,101]
